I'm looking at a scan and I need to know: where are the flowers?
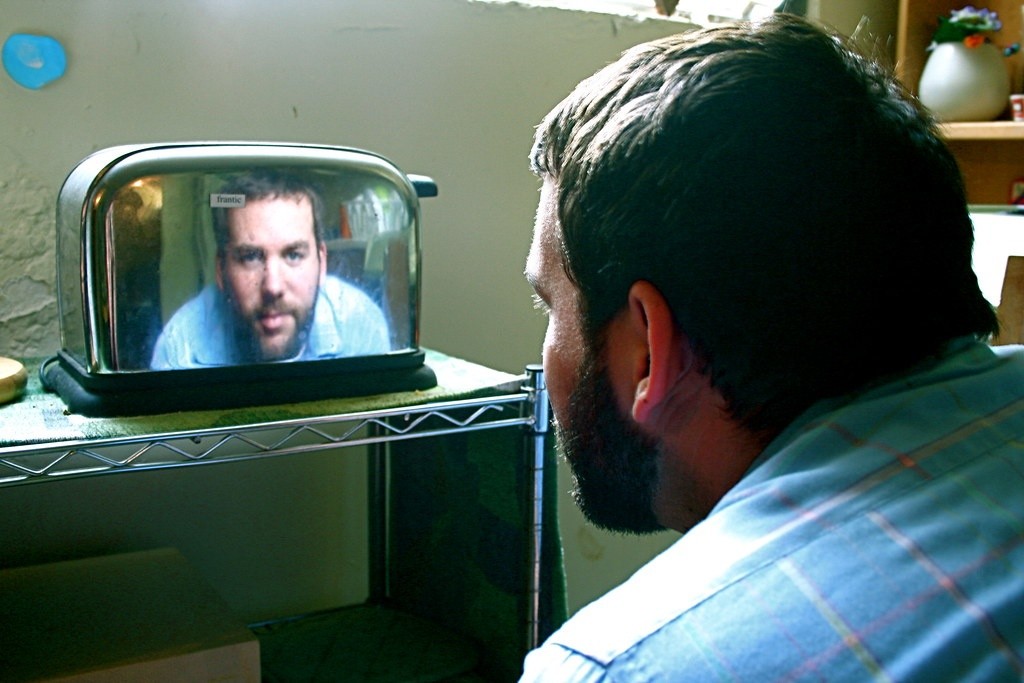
[924,5,1002,52]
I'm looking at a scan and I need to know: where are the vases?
[917,42,1010,123]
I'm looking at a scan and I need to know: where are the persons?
[150,177,391,370]
[519,13,1024,683]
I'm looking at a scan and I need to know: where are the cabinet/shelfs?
[894,0,1024,348]
